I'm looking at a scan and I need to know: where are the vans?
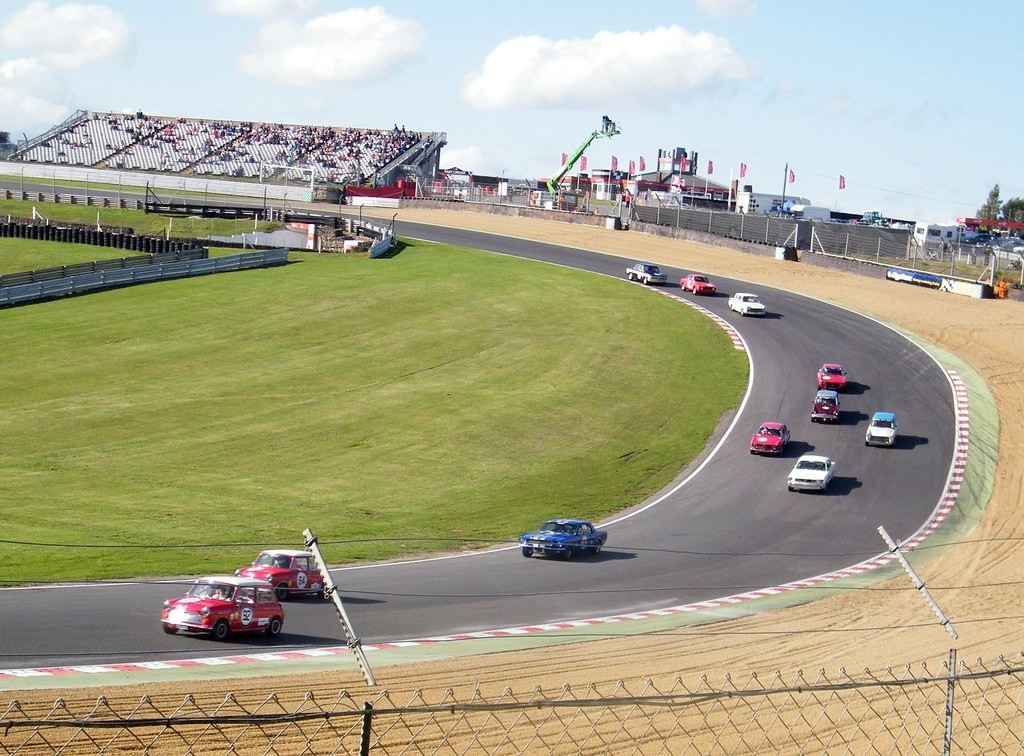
[789,205,831,223]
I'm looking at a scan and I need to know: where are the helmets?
[275,558,285,568]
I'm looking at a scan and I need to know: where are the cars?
[519,519,606,559]
[787,455,835,492]
[751,422,789,456]
[728,293,767,317]
[864,412,898,447]
[236,549,326,600]
[161,576,284,638]
[990,237,1024,254]
[817,363,849,394]
[626,263,667,284]
[812,391,841,423]
[973,247,998,267]
[680,274,717,295]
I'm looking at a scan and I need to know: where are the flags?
[612,156,617,171]
[672,175,685,186]
[581,156,586,171]
[788,169,795,182]
[629,161,635,174]
[840,174,846,189]
[681,156,687,170]
[740,163,746,177]
[671,187,682,194]
[562,154,568,166]
[709,161,713,174]
[640,157,645,171]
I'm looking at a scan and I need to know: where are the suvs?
[962,234,998,249]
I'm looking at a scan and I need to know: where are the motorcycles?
[1006,260,1022,271]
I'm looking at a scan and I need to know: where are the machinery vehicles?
[529,121,621,211]
[860,211,891,228]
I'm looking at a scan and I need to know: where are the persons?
[625,193,630,208]
[212,587,226,600]
[40,109,422,186]
[971,244,976,264]
[594,208,599,214]
[997,277,1006,299]
